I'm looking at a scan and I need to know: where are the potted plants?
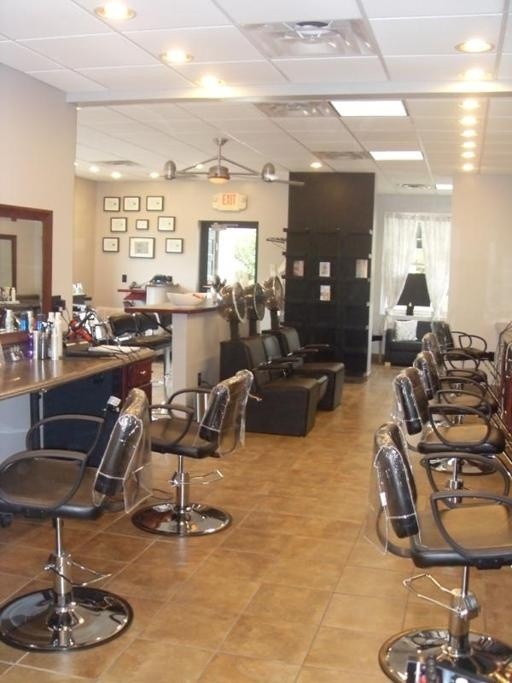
[211,274,227,305]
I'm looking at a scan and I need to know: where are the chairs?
[133,311,171,351]
[109,315,165,371]
[0,388,150,653]
[131,369,254,536]
[158,312,171,328]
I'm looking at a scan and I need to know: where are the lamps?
[162,136,305,187]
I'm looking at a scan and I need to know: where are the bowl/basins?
[167,291,208,306]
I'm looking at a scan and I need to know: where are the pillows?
[391,320,418,341]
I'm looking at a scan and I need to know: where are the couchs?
[262,326,344,411]
[384,319,453,366]
[256,334,328,403]
[219,336,319,437]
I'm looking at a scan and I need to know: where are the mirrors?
[0,205,53,345]
[1,234,17,298]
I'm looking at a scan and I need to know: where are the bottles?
[4,286,16,302]
[3,310,63,361]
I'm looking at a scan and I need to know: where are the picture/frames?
[102,195,183,260]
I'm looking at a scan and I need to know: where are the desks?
[0,346,155,468]
[124,290,272,418]
